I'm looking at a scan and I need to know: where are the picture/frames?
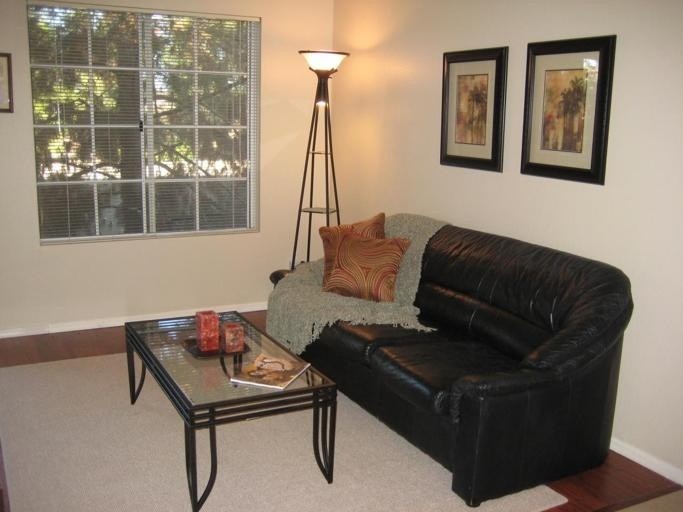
[0,53,15,113]
[440,35,618,185]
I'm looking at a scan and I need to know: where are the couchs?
[269,212,634,507]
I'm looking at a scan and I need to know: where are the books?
[230,352,312,391]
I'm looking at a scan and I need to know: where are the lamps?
[287,51,350,272]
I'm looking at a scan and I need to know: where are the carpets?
[1,351,570,512]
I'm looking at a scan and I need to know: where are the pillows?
[320,212,412,303]
